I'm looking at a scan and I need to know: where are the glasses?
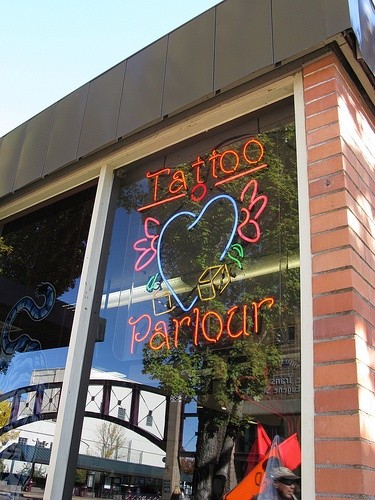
[277,478,297,486]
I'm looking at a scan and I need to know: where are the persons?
[250,456,301,500]
[170,485,183,500]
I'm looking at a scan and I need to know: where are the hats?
[271,467,300,480]
